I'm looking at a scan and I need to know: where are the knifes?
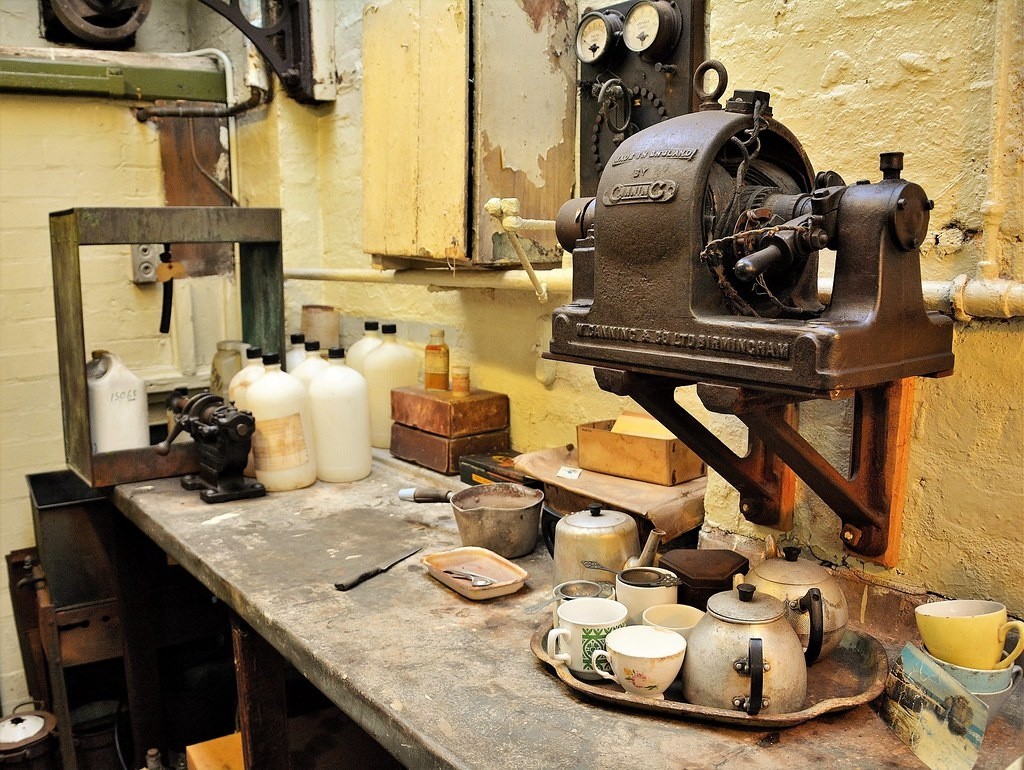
[334,546,422,591]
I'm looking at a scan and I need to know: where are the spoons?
[443,568,494,586]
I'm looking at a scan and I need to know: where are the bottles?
[211,321,420,492]
[425,330,449,390]
[176,754,187,770]
[146,748,166,770]
[86,350,150,455]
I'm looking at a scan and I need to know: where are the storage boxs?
[576,418,707,486]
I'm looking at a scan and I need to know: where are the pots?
[398,483,544,559]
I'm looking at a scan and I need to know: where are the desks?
[108,444,1024,770]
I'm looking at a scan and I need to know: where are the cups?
[548,597,628,680]
[643,604,705,640]
[615,567,677,626]
[915,600,1024,732]
[592,625,687,700]
[553,580,616,628]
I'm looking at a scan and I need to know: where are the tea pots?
[541,502,666,589]
[744,533,848,668]
[683,573,808,716]
